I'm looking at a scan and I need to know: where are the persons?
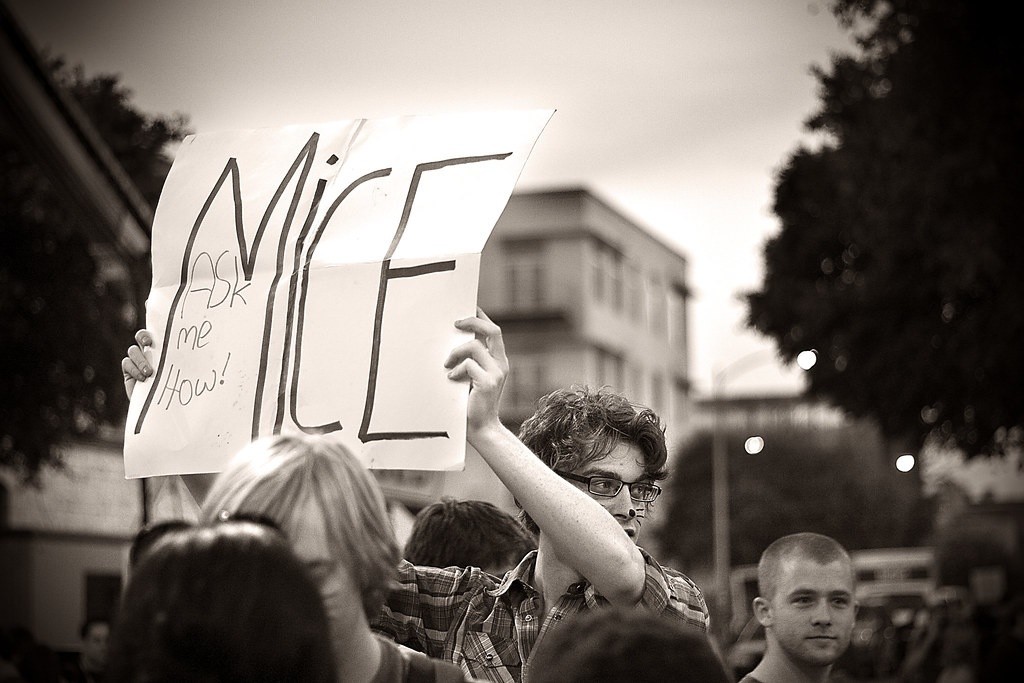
[404,498,538,580]
[197,434,497,683]
[104,512,338,683]
[122,305,710,683]
[0,619,112,683]
[739,532,860,683]
[897,566,1024,683]
[524,606,733,683]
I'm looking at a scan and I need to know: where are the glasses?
[554,469,662,502]
[129,506,283,574]
